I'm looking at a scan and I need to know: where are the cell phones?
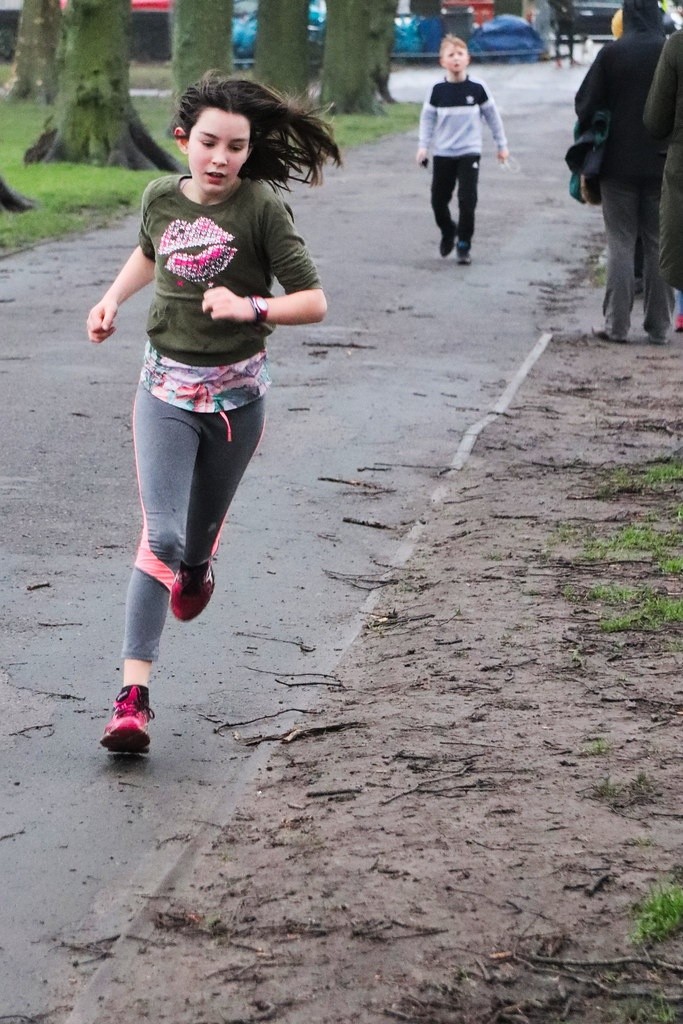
[420,157,429,168]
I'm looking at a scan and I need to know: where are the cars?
[551,2,621,37]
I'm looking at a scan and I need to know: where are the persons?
[642,0,681,334]
[415,27,512,269]
[83,71,346,761]
[572,0,676,349]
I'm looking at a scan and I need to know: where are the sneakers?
[455,240,472,264]
[169,562,215,620]
[100,686,150,753]
[439,223,456,255]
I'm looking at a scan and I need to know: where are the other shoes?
[673,313,683,331]
[592,326,626,342]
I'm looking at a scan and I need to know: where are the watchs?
[242,293,270,328]
